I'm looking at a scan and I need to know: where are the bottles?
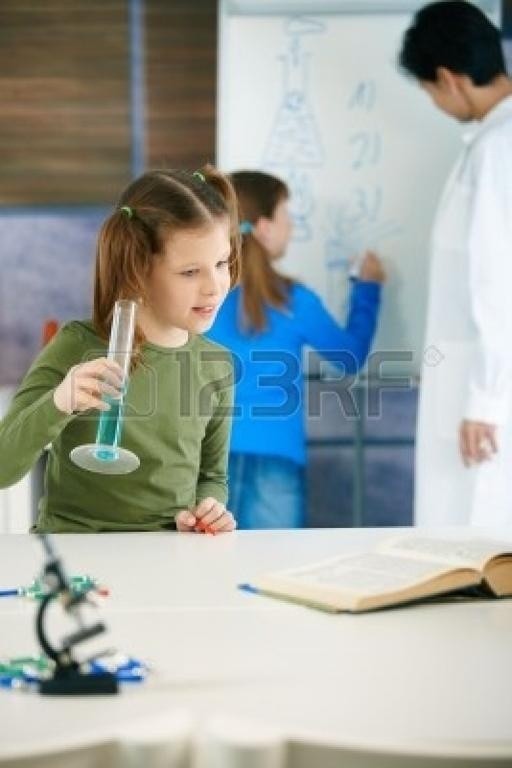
[68,296,144,478]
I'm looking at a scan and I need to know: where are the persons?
[0,165,243,535]
[398,2,510,530]
[202,172,384,527]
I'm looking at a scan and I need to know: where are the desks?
[0,524,512,768]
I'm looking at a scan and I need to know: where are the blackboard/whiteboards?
[215,0,504,392]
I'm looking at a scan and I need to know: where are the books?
[238,536,511,613]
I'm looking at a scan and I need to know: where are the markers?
[349,252,366,282]
